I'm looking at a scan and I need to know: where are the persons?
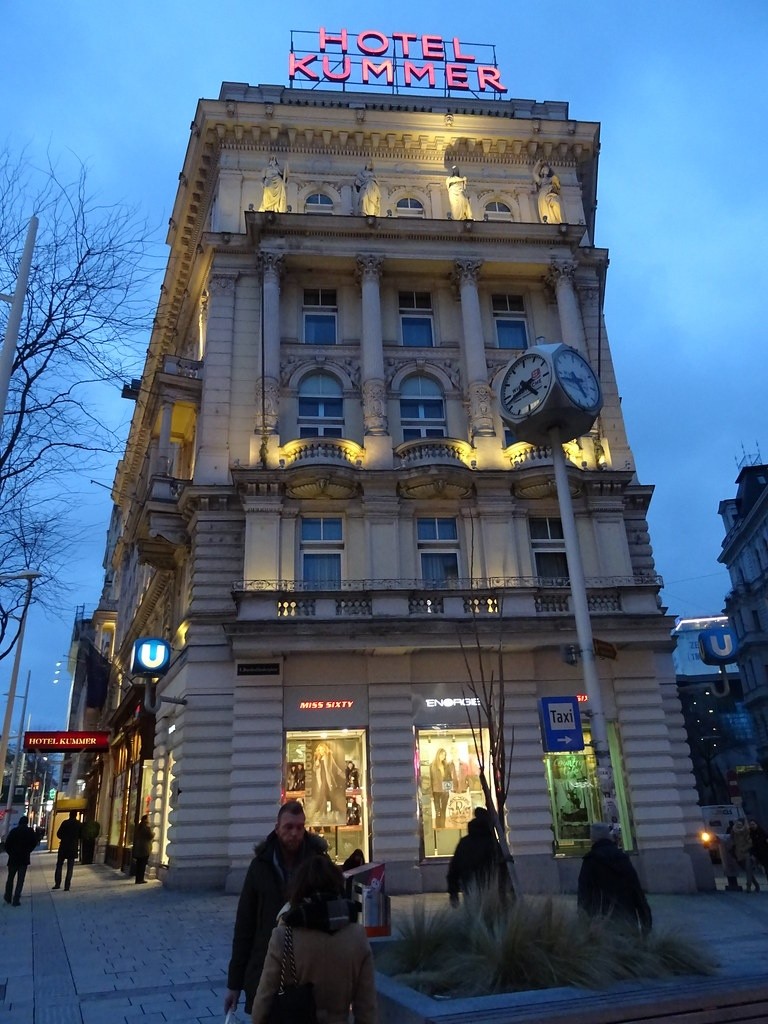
[577,823,653,946]
[133,815,155,884]
[346,798,362,826]
[312,744,346,817]
[224,800,328,1016]
[431,748,450,828]
[52,812,83,891]
[346,759,359,789]
[287,764,305,790]
[448,806,517,923]
[3,817,38,906]
[726,819,768,893]
[251,853,375,1024]
[447,746,470,793]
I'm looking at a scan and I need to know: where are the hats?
[591,823,612,842]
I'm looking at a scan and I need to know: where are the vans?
[701,805,750,851]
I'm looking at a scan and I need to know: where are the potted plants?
[80,821,100,865]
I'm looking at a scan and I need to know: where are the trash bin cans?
[341,863,388,938]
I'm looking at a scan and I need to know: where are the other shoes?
[755,886,760,895]
[3,893,12,904]
[13,901,21,906]
[746,886,752,893]
[52,886,60,890]
[64,889,69,891]
[135,880,147,884]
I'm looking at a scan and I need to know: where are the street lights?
[2,669,33,839]
[0,571,42,791]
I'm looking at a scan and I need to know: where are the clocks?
[498,352,552,419]
[554,350,602,411]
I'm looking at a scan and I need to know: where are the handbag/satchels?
[261,978,319,1024]
